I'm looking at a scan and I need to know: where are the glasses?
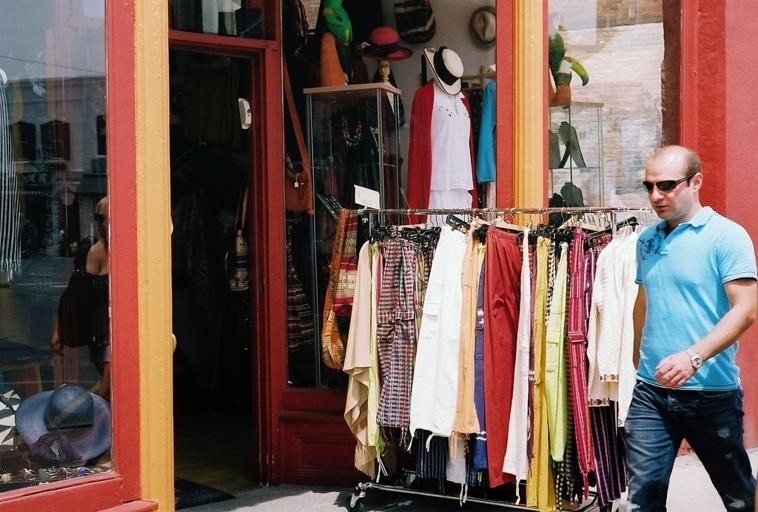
[93,213,106,223]
[642,175,694,191]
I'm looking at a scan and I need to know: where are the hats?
[362,27,412,60]
[469,5,496,49]
[423,46,464,95]
[15,384,112,466]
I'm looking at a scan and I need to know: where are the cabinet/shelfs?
[546,101,608,203]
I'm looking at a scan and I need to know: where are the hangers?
[360,204,650,257]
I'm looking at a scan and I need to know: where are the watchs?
[684,345,705,371]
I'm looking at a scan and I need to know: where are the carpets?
[171,474,236,508]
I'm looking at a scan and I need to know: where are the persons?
[48,195,110,441]
[405,45,478,228]
[617,144,757,512]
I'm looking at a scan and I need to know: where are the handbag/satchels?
[225,189,249,292]
[285,161,314,215]
